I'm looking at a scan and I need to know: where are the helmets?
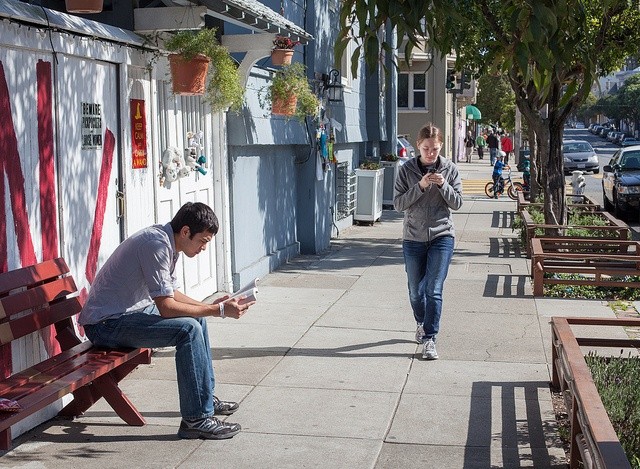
[496,151,507,158]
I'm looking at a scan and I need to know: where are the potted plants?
[164,26,246,115]
[264,63,320,124]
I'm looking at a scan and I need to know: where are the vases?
[63,0,102,15]
[270,44,294,68]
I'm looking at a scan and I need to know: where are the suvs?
[600,128,611,138]
[621,137,640,147]
[618,134,634,147]
[606,131,615,142]
[613,133,623,144]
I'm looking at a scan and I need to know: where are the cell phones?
[427,168,438,173]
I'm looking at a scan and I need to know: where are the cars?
[561,139,599,175]
[601,145,640,218]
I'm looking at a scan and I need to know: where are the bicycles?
[510,174,531,198]
[485,166,523,200]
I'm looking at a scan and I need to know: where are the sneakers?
[422,338,438,359]
[177,416,242,439]
[415,321,425,344]
[212,395,239,415]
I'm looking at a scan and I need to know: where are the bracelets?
[219,302,224,318]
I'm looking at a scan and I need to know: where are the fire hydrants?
[569,170,586,202]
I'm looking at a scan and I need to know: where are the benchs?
[1,256,154,450]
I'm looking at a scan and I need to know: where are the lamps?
[322,68,345,102]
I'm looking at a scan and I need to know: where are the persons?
[492,151,506,199]
[519,154,531,191]
[486,130,499,166]
[76,202,256,440]
[501,133,513,165]
[464,131,475,163]
[476,132,486,159]
[392,124,464,361]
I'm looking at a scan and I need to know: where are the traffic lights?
[461,68,472,89]
[445,69,457,90]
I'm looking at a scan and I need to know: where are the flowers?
[273,35,300,49]
